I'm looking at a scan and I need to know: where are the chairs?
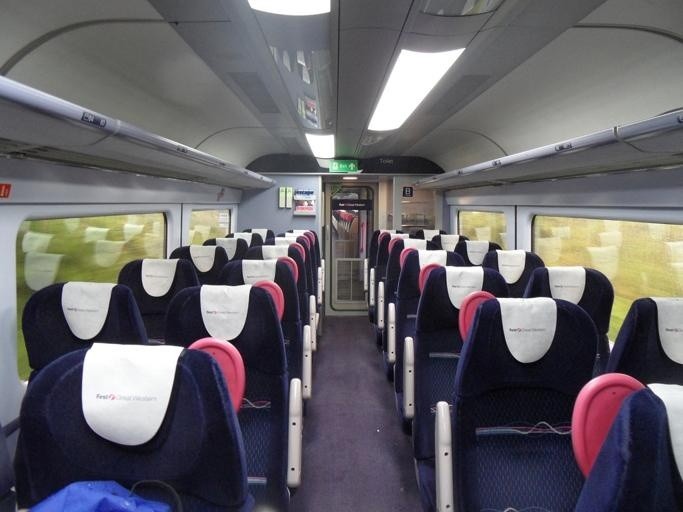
[333,211,357,236]
